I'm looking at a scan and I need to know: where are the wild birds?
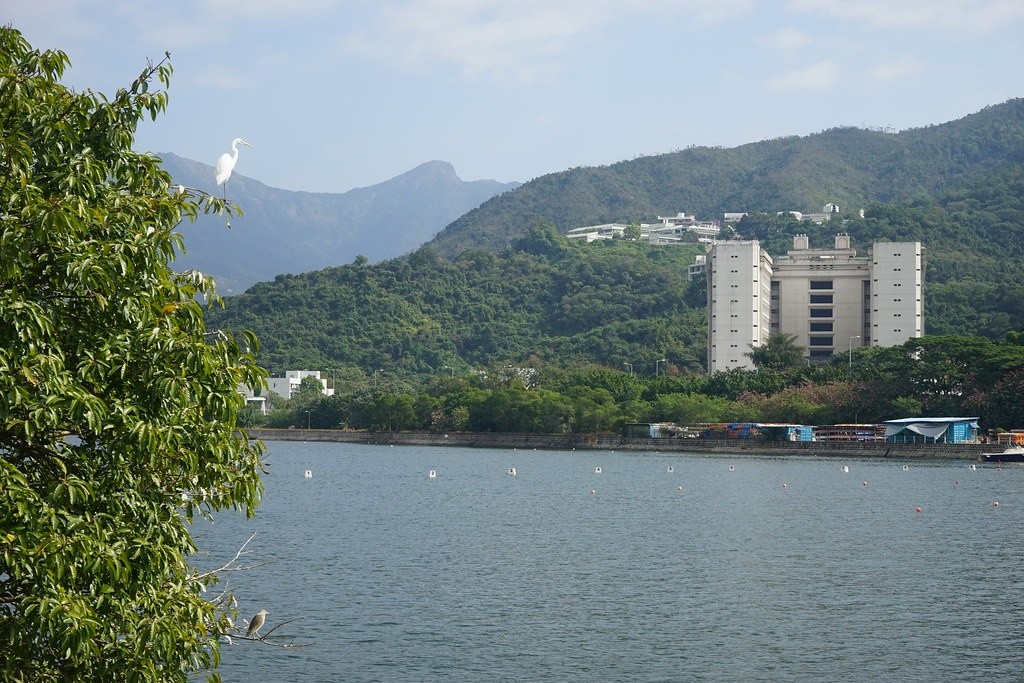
[215,137,252,199]
[246,610,270,641]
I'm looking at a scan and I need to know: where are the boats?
[981,444,1024,463]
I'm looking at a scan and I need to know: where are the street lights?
[627,364,632,376]
[656,359,665,376]
[849,336,860,373]
[374,369,384,388]
[326,369,335,396]
[444,366,453,378]
[305,411,310,429]
